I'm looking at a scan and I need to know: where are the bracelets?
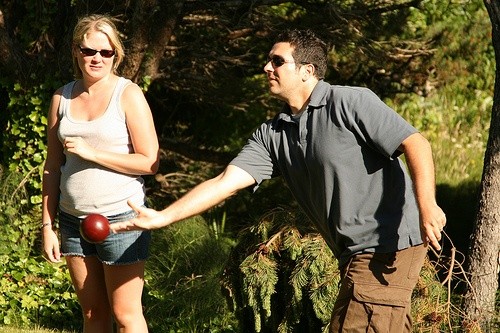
[41,223,52,228]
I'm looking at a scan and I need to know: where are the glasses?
[76,44,115,58]
[267,55,317,68]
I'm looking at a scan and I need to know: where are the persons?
[41,14,160,333]
[110,29,446,332]
